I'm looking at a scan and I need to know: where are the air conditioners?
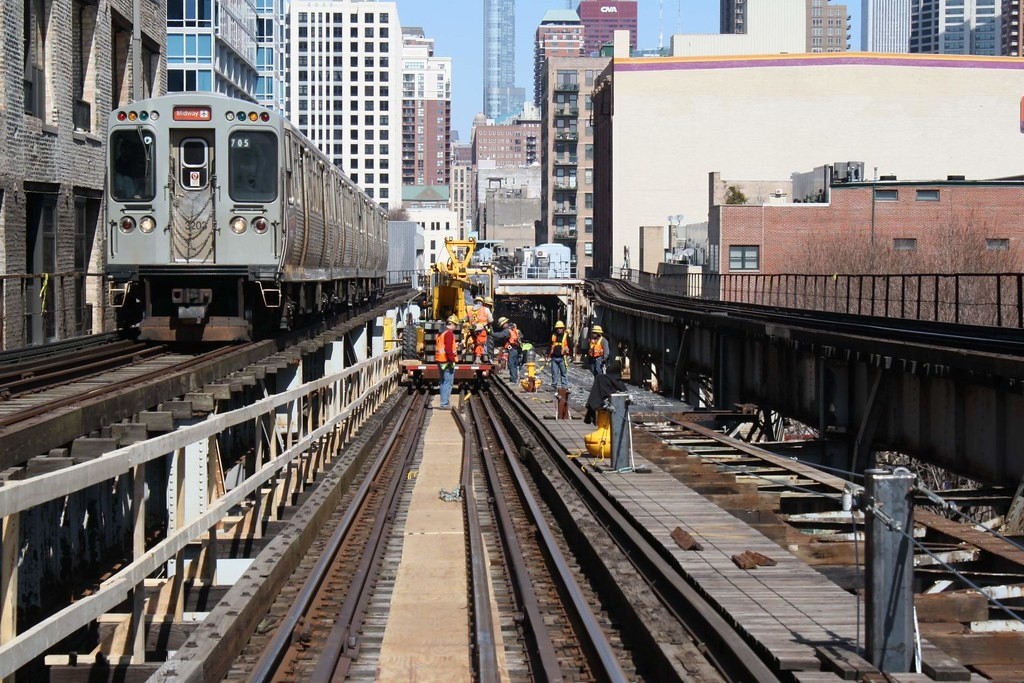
[534,248,548,258]
[775,188,783,195]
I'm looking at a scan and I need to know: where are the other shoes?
[441,406,452,411]
[546,387,556,391]
[506,382,515,386]
[475,359,482,363]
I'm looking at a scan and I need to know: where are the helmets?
[498,317,509,327]
[474,297,483,303]
[555,321,565,328]
[592,326,603,333]
[447,315,458,324]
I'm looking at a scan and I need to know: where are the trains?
[101,90,393,349]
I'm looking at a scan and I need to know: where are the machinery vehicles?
[398,234,502,393]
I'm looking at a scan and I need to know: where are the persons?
[546,321,573,394]
[588,325,609,377]
[485,316,524,384]
[435,315,459,410]
[464,297,493,365]
[585,361,628,426]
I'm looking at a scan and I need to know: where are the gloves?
[602,357,607,364]
[449,362,454,374]
